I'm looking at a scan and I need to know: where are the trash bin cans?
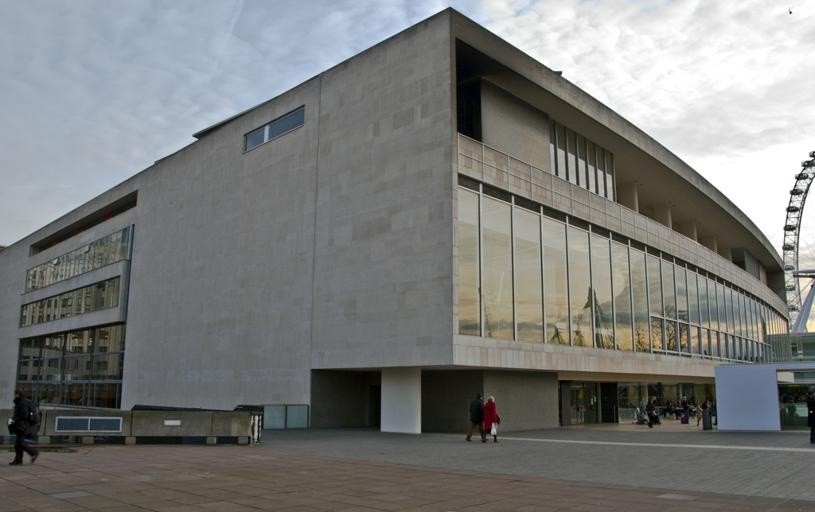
[703,408,712,430]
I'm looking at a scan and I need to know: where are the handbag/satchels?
[491,423,499,436]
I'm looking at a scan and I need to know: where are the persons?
[5,389,40,466]
[633,391,716,430]
[465,392,486,442]
[806,389,815,445]
[482,396,502,444]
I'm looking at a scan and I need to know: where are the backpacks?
[18,399,39,425]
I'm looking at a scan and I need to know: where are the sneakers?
[9,452,40,465]
